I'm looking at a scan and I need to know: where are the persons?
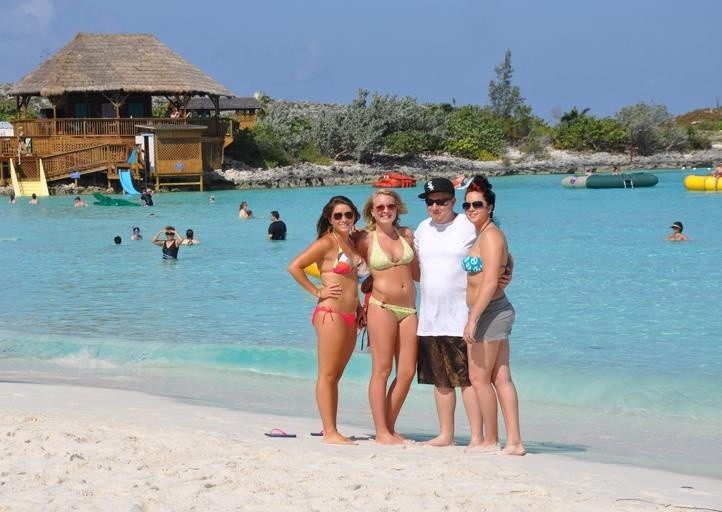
[73,197,87,208]
[665,222,688,241]
[353,188,420,445]
[612,167,619,175]
[29,193,39,205]
[462,174,526,456]
[17,141,28,165]
[9,195,16,204]
[113,189,199,261]
[585,168,592,176]
[268,211,287,241]
[209,195,215,203]
[351,177,513,450]
[239,201,248,219]
[712,162,722,188]
[288,196,367,446]
[247,211,253,218]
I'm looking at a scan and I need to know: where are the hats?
[670,221,683,230]
[417,178,454,199]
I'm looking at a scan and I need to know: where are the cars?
[0,120,23,140]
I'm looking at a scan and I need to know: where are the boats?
[370,171,416,190]
[449,170,472,192]
[684,174,722,192]
[563,173,660,190]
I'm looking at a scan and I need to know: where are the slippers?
[264,428,297,439]
[311,427,337,436]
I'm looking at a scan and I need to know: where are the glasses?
[331,212,355,220]
[374,204,396,210]
[462,200,488,209]
[424,196,452,206]
